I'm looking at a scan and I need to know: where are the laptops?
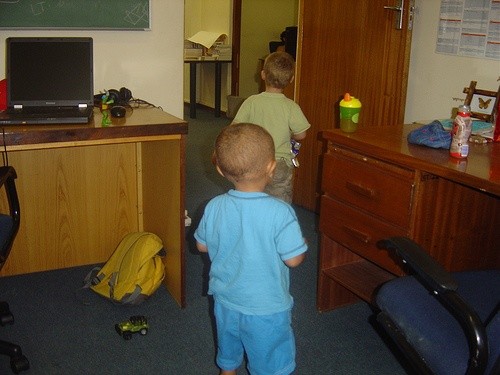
[0,36,94,123]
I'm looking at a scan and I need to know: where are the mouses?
[110,105,126,119]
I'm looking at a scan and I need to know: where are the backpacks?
[82,232,167,308]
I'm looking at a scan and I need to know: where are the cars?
[115,315,150,340]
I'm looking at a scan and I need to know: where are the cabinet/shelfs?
[318,122,500,313]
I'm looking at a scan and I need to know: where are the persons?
[193,123,309,375]
[211,51,311,205]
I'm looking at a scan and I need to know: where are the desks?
[0,102,189,312]
[184,55,232,119]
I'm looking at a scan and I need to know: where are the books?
[184,31,231,59]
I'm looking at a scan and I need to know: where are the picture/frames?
[462,81,500,121]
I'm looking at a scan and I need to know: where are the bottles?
[450,105,472,158]
[339,92,362,133]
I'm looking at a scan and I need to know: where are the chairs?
[0,166,31,375]
[366,237,500,375]
